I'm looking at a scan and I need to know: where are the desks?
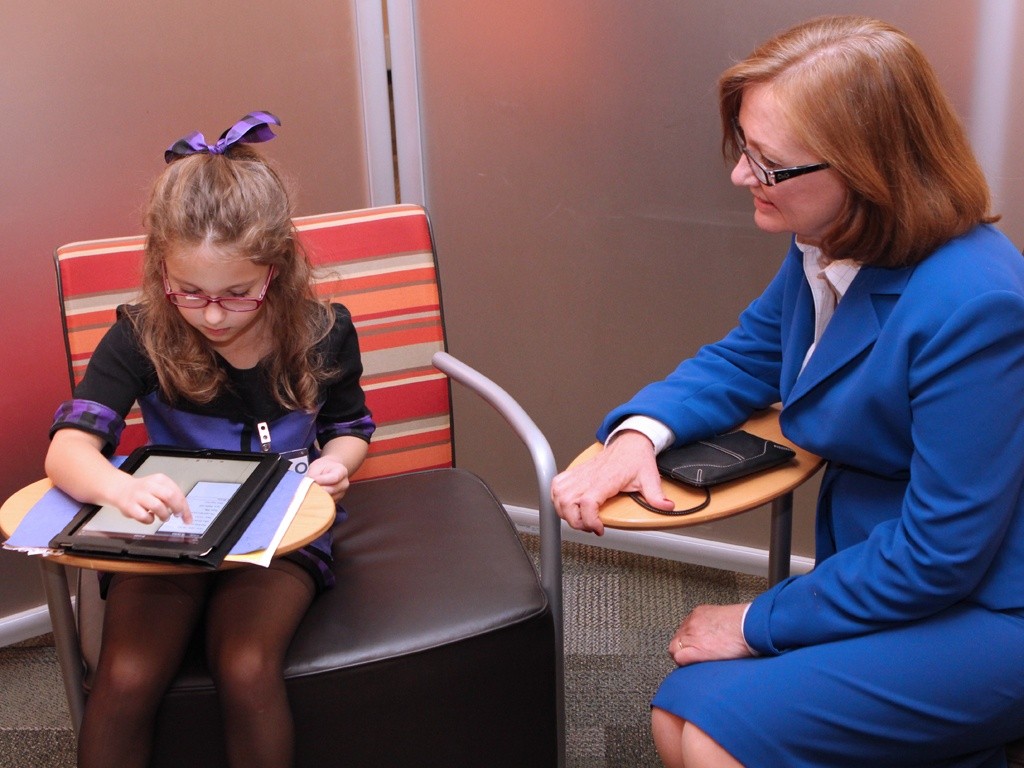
[561,391,831,597]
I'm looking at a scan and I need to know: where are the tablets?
[47,445,294,569]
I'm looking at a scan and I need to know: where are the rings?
[678,638,684,650]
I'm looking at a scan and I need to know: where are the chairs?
[0,202,570,767]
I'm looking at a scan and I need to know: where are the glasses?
[728,115,831,186]
[157,259,275,313]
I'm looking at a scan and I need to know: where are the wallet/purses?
[626,427,797,517]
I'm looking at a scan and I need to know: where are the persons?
[549,9,1024,768]
[42,107,376,768]
